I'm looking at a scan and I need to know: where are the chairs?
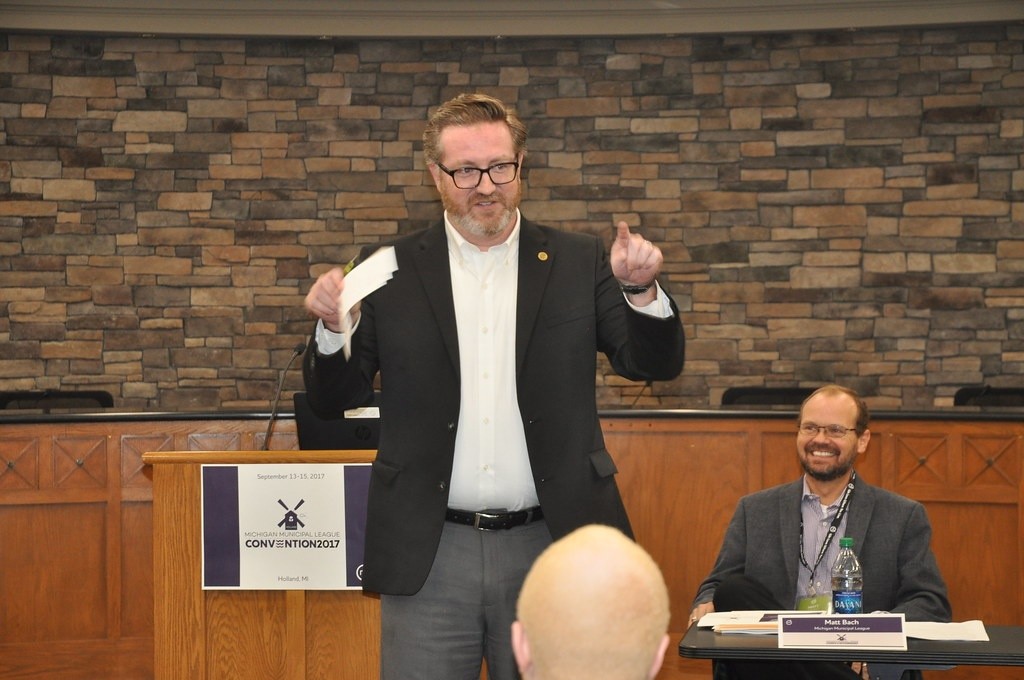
[0,391,113,408]
[955,387,1024,408]
[722,387,817,406]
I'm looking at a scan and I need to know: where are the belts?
[445,505,544,532]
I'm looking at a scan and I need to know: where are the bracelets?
[614,276,656,294]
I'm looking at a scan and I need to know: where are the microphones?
[632,380,653,406]
[261,342,305,452]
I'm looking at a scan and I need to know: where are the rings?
[691,617,696,620]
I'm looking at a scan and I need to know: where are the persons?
[688,385,953,680]
[307,94,685,679]
[512,525,671,678]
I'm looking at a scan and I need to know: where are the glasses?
[438,154,519,189]
[797,423,856,438]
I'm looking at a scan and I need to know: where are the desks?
[678,622,1024,680]
[0,407,1024,679]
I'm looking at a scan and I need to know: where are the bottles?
[831,538,863,614]
